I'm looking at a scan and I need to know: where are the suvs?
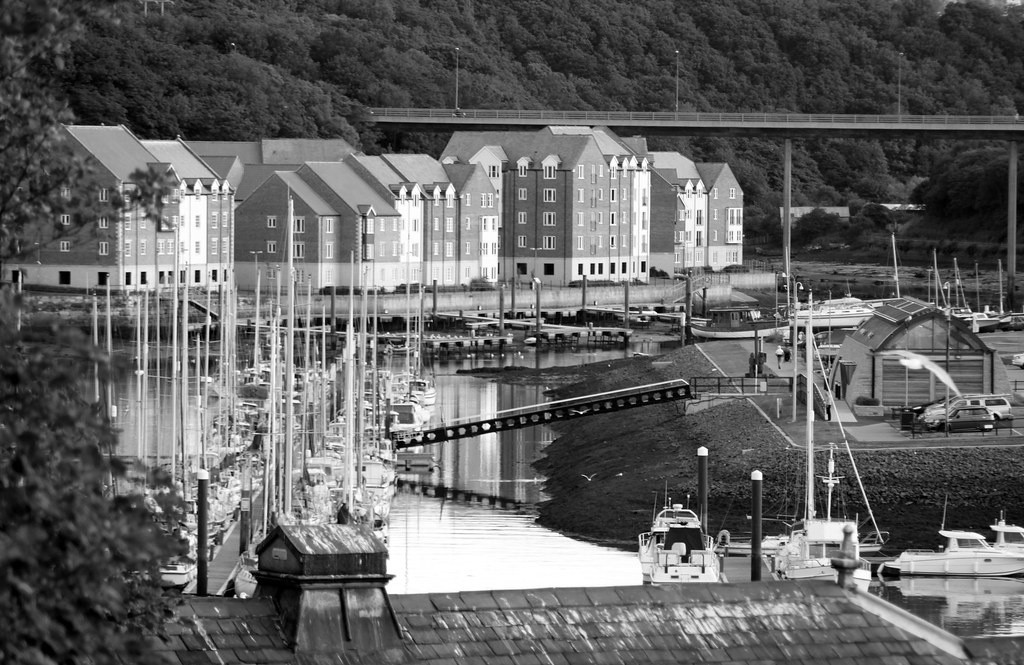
[917,394,1013,428]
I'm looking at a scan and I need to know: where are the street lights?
[898,52,904,116]
[674,50,679,112]
[454,48,459,109]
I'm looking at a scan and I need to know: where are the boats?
[874,495,1024,580]
[638,475,727,587]
[690,271,792,340]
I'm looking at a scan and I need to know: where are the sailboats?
[780,232,1024,337]
[760,294,875,598]
[70,181,426,587]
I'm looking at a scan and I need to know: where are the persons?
[821,388,833,422]
[775,344,785,368]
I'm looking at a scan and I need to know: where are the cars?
[924,404,996,431]
[1012,352,1024,368]
